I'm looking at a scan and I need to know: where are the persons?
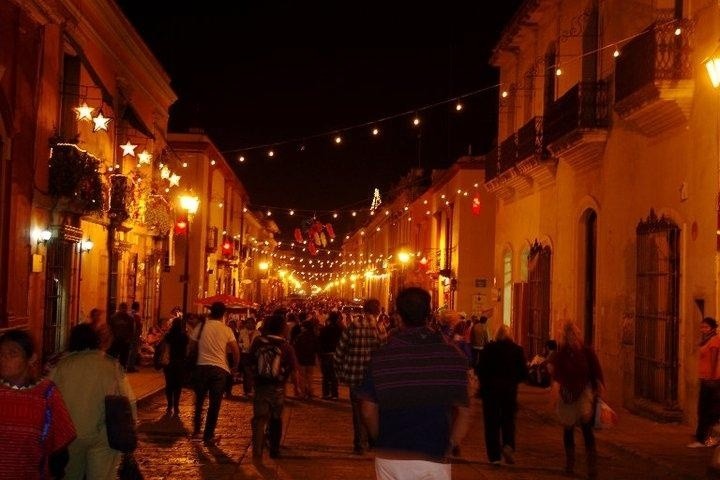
[86,292,557,459]
[477,324,527,469]
[682,318,720,450]
[357,289,475,479]
[547,318,605,480]
[1,327,77,479]
[48,322,139,480]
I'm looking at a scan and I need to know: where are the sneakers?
[687,441,705,448]
[503,445,516,464]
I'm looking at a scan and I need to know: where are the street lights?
[397,249,411,292]
[179,190,201,321]
[253,260,375,301]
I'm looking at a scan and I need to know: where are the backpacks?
[256,335,290,383]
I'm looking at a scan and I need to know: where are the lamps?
[62,78,115,134]
[82,235,95,253]
[702,37,720,89]
[118,124,154,164]
[35,230,51,249]
[158,152,183,189]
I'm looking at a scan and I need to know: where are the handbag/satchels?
[153,342,171,369]
[104,394,138,452]
[186,342,198,372]
